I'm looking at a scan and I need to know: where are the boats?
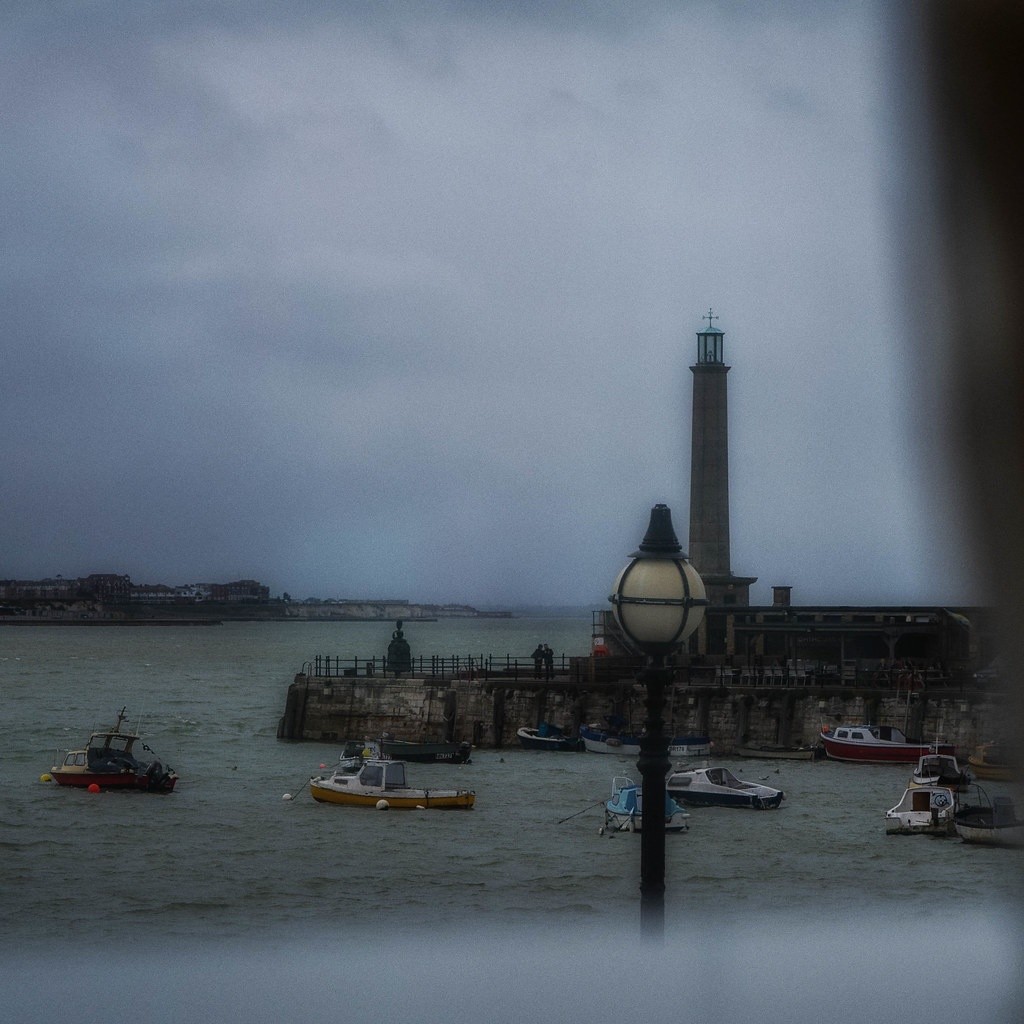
[606,776,690,833]
[820,723,956,765]
[581,726,713,757]
[734,741,820,760]
[953,797,1024,848]
[342,734,473,764]
[665,766,784,810]
[907,752,971,792]
[967,740,1024,782]
[51,704,180,792]
[518,726,583,753]
[886,786,956,835]
[309,758,476,809]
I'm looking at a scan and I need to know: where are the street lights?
[613,505,707,948]
